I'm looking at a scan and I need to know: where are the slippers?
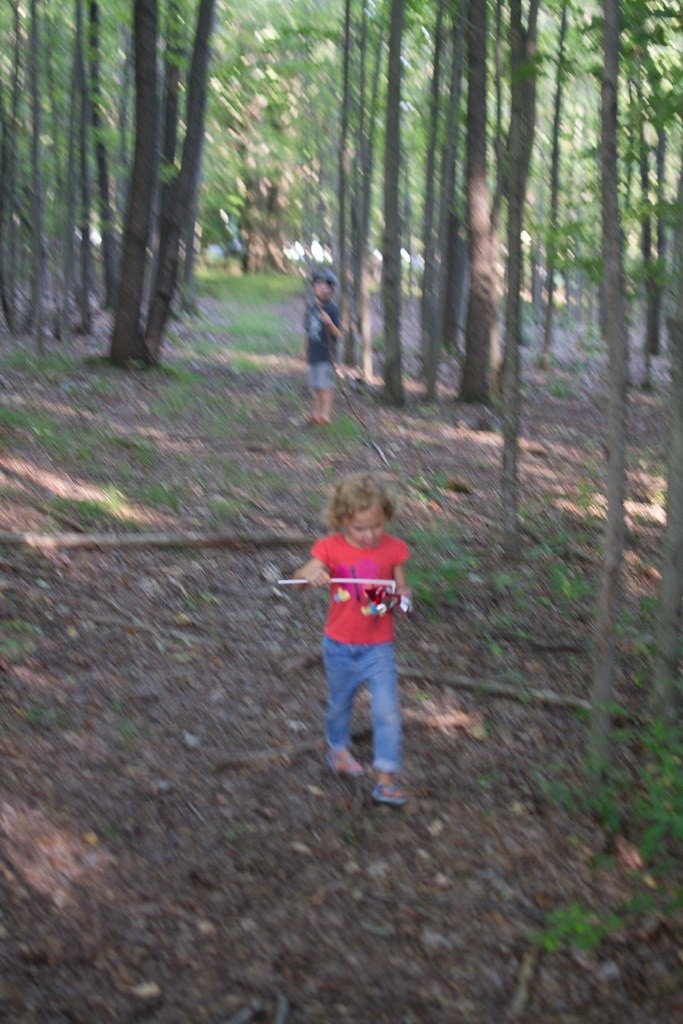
[373,782,407,806]
[326,749,364,778]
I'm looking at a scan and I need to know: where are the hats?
[311,268,340,288]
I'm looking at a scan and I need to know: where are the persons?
[303,269,344,425]
[293,473,414,803]
